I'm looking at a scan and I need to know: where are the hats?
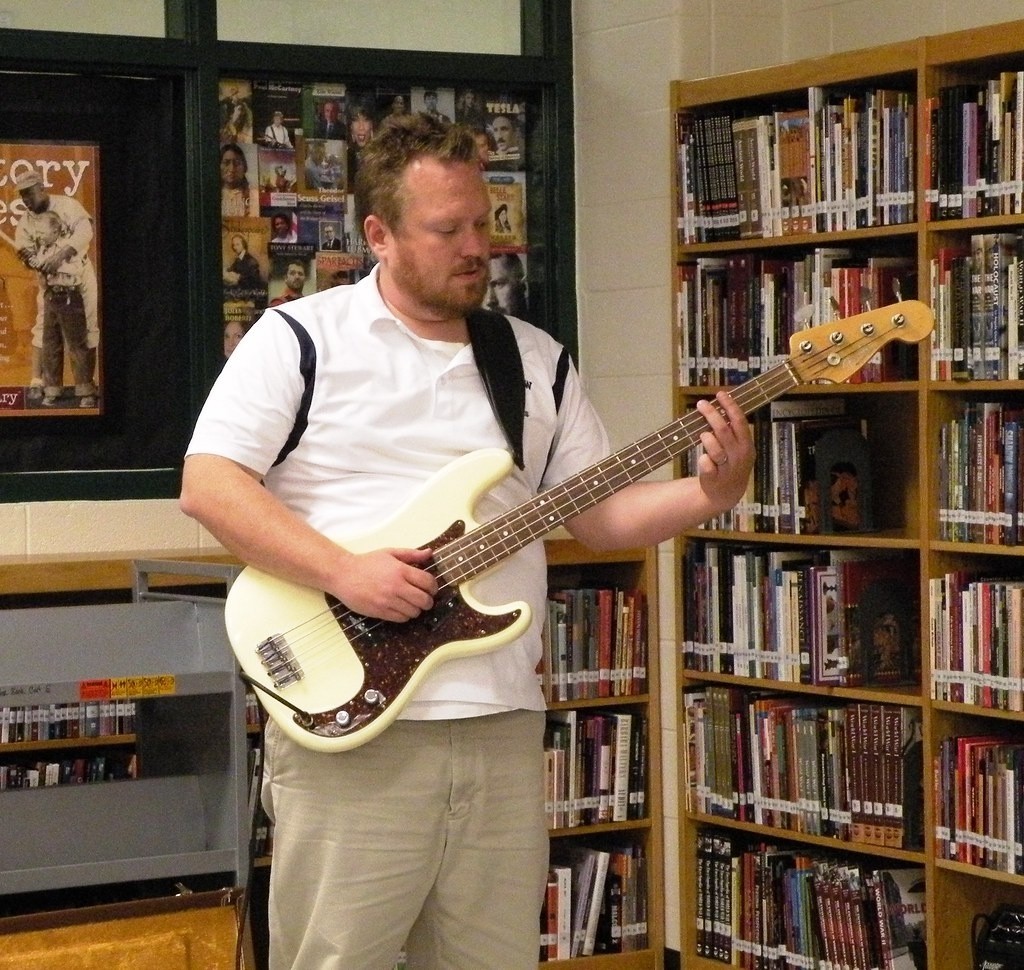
[12,170,42,193]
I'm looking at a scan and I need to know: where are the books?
[0,138,103,415]
[244,691,274,855]
[0,702,142,787]
[538,577,651,961]
[677,68,1024,970]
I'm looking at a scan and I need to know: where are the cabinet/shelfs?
[0,527,665,970]
[0,558,257,970]
[666,22,1024,970]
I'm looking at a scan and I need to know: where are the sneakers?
[28,387,41,398]
[40,396,58,407]
[78,396,94,406]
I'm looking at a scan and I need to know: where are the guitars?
[225,298,936,757]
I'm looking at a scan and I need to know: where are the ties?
[329,241,332,248]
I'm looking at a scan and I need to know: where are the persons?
[181,106,755,970]
[221,69,531,366]
[15,170,99,408]
